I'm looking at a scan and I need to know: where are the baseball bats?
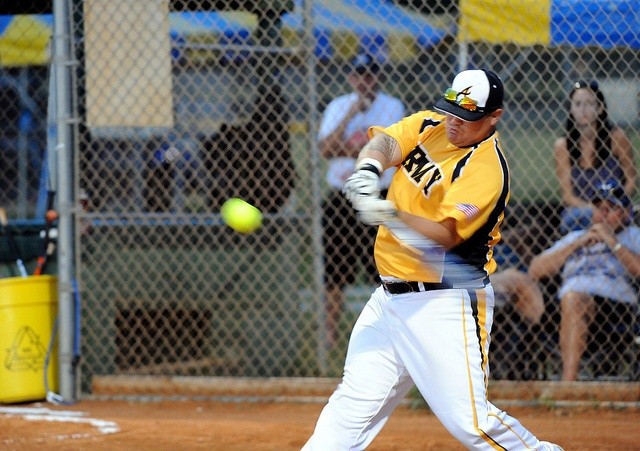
[344,182,488,290]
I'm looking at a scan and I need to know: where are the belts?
[379,275,489,295]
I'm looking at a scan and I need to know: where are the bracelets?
[610,243,622,255]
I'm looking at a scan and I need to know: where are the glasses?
[575,79,599,91]
[443,88,486,112]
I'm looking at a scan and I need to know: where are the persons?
[501,179,640,381]
[315,52,407,350]
[300,70,565,451]
[553,79,637,235]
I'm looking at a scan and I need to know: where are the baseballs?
[220,197,263,234]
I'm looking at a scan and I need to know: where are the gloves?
[342,157,398,226]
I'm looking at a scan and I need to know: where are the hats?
[432,69,504,123]
[592,180,630,206]
